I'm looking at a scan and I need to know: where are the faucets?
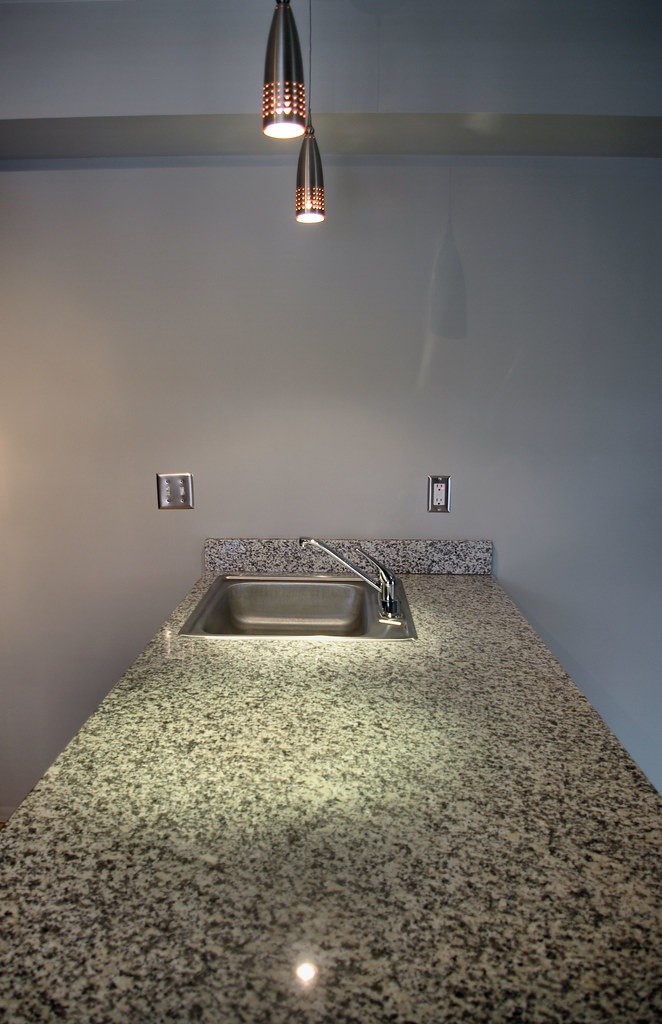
[298,536,404,620]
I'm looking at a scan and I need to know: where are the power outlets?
[426,475,453,514]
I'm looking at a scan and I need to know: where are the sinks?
[176,573,418,642]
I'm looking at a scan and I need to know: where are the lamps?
[295,0,326,224]
[262,0,306,140]
